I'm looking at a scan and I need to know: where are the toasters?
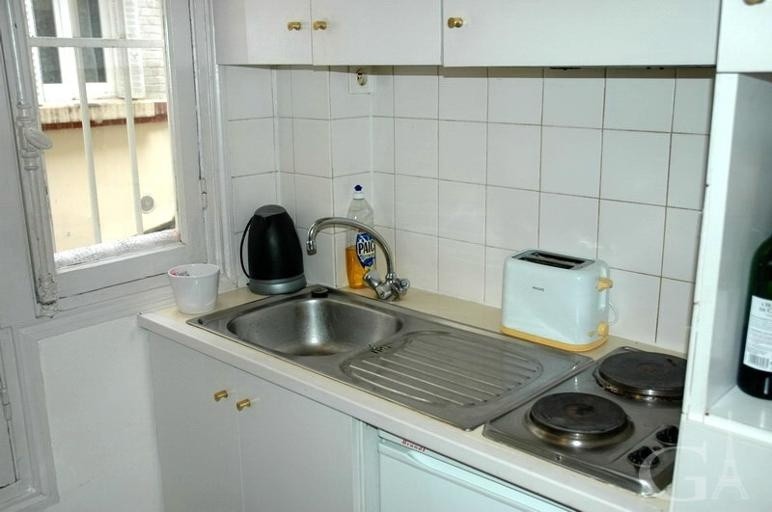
[499,249,612,351]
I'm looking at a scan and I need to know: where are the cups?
[168,263,221,316]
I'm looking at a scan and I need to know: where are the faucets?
[305,216,409,302]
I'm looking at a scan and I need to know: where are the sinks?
[188,284,405,358]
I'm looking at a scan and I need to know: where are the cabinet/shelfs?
[442,3,721,68]
[669,1,770,511]
[145,332,542,512]
[215,0,444,67]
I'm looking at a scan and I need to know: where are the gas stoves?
[482,346,686,495]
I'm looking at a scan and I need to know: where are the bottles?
[734,234,772,402]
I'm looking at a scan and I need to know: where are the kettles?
[239,203,308,296]
